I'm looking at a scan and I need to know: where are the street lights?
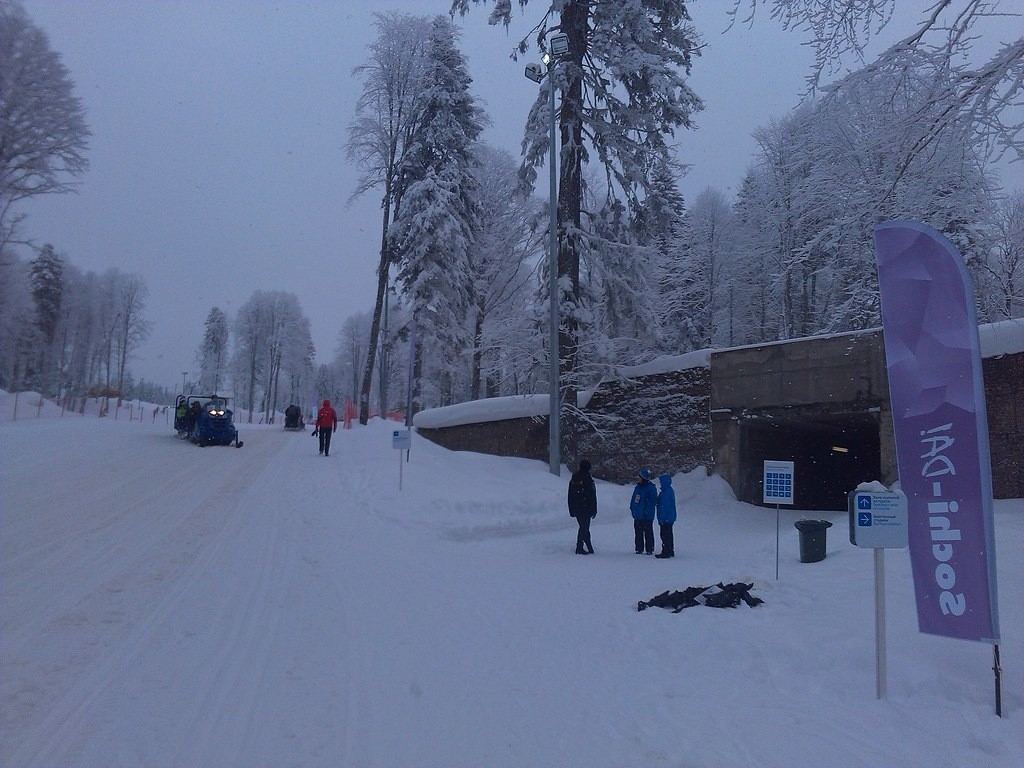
[180,371,189,395]
[524,32,571,481]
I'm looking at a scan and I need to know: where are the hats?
[180,396,186,403]
[639,469,651,480]
[194,401,200,406]
[580,460,591,471]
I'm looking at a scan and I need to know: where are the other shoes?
[636,550,642,554]
[646,551,652,555]
[319,449,323,454]
[325,451,328,456]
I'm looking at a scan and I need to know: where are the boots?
[586,542,594,553]
[575,542,588,555]
[655,543,669,558]
[668,544,674,556]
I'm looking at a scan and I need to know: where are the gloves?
[312,430,318,437]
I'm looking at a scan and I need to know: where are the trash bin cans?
[793,520,833,563]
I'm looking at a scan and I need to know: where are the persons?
[629,470,677,558]
[311,400,337,456]
[568,460,597,555]
[177,395,223,431]
